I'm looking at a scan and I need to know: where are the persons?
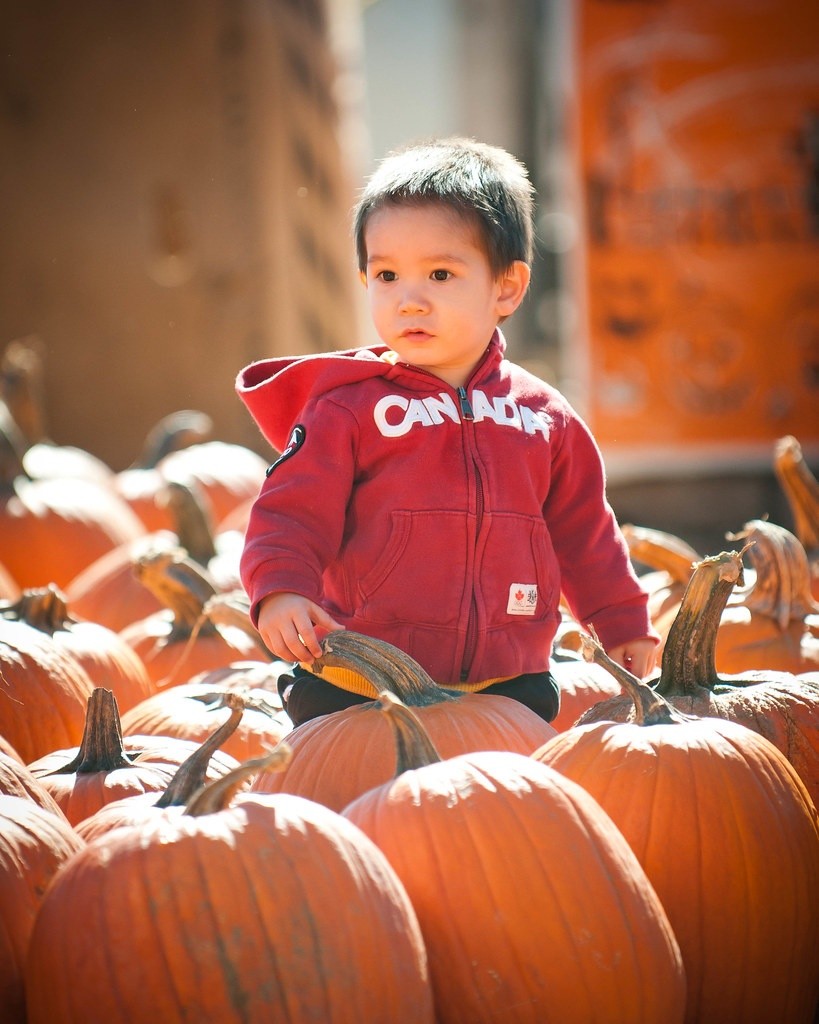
[227,134,661,726]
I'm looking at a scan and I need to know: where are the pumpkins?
[0,337,819,1024]
